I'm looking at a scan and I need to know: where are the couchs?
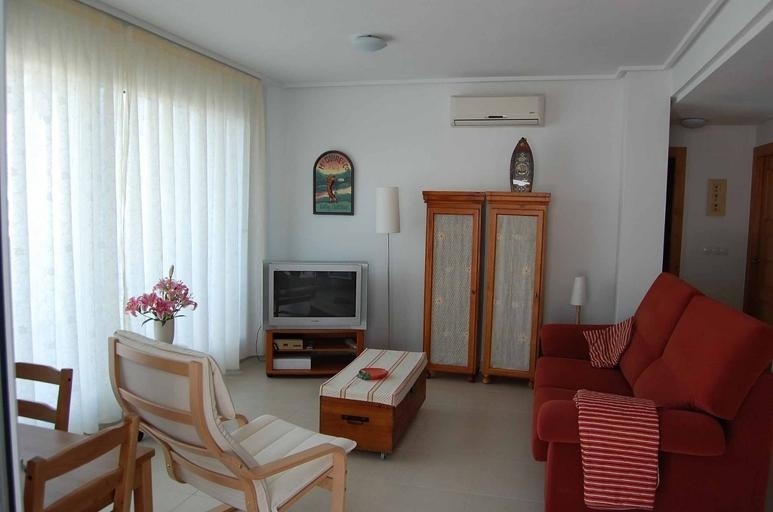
[533,274,773,512]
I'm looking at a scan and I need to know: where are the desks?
[16,422,156,511]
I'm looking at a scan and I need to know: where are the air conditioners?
[450,94,545,128]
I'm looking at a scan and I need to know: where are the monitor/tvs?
[262,259,369,330]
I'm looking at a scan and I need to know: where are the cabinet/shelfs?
[265,328,363,376]
[421,191,550,389]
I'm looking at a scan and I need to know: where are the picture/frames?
[313,151,354,217]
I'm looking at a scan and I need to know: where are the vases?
[154,319,175,344]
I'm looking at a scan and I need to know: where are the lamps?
[571,276,587,325]
[374,187,401,350]
[353,36,387,53]
[681,118,711,131]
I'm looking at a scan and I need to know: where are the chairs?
[14,360,74,433]
[24,413,137,512]
[107,330,354,512]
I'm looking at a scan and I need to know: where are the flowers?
[124,265,198,325]
